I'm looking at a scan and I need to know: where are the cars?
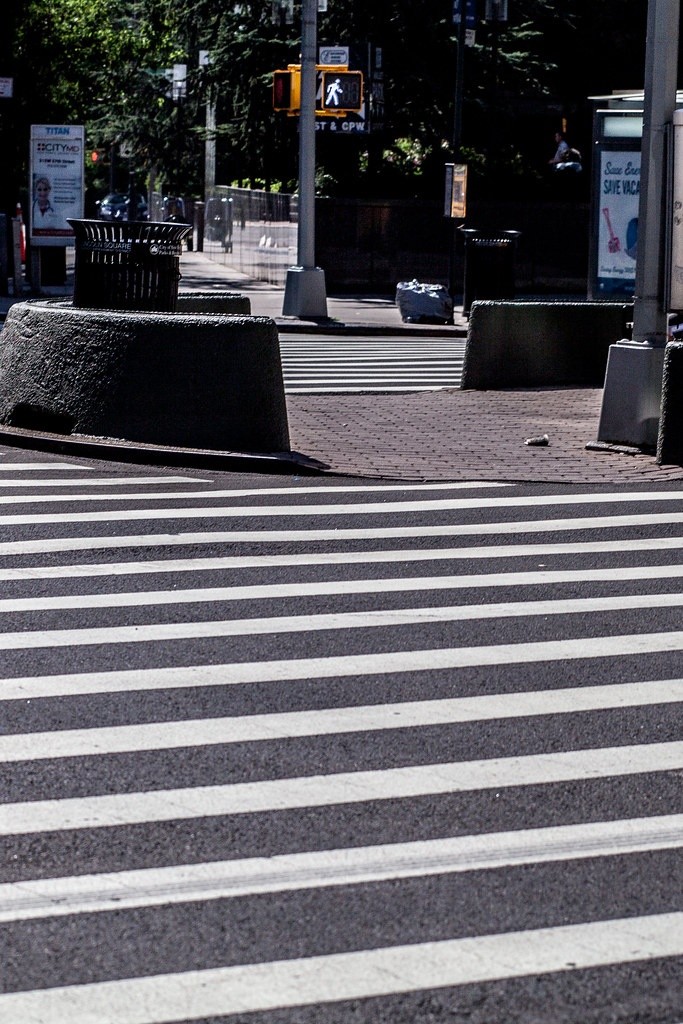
[94,192,150,221]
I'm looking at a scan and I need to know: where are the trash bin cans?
[65,216,193,310]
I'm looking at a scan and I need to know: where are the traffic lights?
[273,68,301,113]
[320,71,365,114]
[84,148,105,170]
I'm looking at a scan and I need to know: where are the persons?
[83,177,99,218]
[32,177,59,229]
[163,185,204,252]
[547,130,582,177]
